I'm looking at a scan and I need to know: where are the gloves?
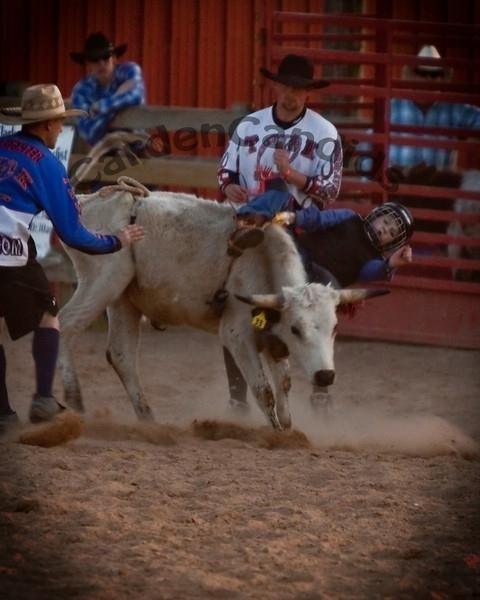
[272,211,295,226]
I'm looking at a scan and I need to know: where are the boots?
[227,212,272,258]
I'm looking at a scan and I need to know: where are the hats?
[0,83,89,125]
[71,32,128,65]
[258,55,331,89]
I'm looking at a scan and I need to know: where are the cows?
[51,130,391,434]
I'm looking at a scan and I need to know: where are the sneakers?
[0,410,22,434]
[29,393,66,425]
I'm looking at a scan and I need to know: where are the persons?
[215,54,415,417]
[352,42,479,249]
[0,33,147,425]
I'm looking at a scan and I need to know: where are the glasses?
[89,53,111,63]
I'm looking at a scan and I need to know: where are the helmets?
[363,201,415,253]
